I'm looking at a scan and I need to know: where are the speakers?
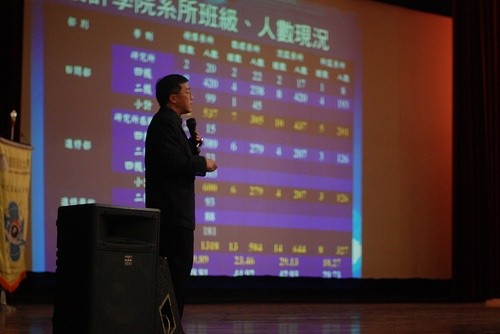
[53,203,162,334]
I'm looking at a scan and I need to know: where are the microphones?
[10,110,17,126]
[186,118,197,137]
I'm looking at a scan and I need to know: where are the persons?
[145,74,218,321]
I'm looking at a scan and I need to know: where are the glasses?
[179,90,192,97]
